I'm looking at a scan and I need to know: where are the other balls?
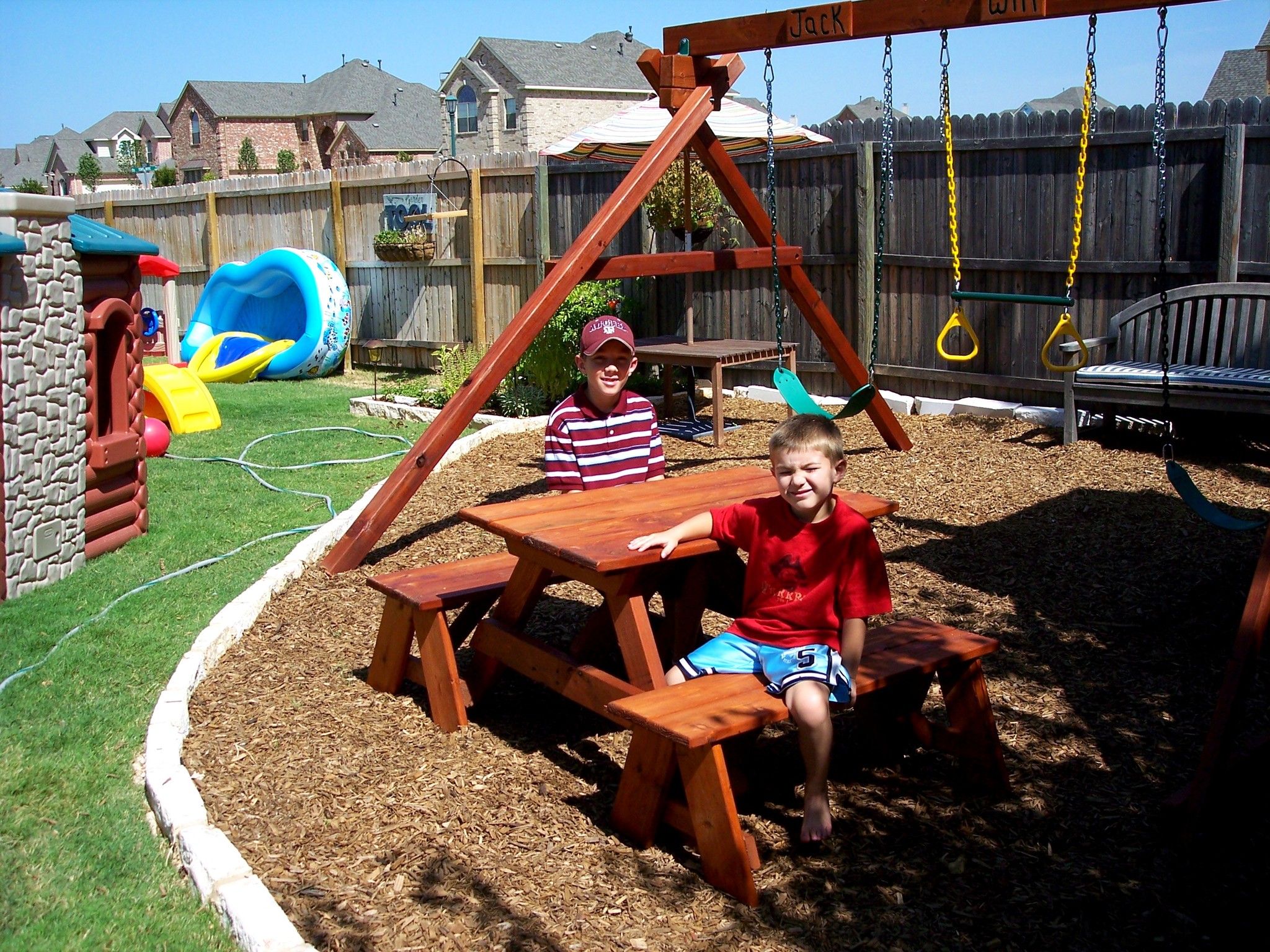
[142,417,171,460]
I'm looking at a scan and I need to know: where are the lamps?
[362,340,388,401]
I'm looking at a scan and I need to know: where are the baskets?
[668,226,713,243]
[374,243,435,261]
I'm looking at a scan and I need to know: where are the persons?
[627,414,893,841]
[543,316,714,668]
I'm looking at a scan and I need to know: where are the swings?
[761,29,895,427]
[936,30,1098,373]
[1151,5,1270,538]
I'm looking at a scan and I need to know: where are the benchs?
[366,551,1013,908]
[1059,282,1270,449]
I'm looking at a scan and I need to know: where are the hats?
[581,316,635,357]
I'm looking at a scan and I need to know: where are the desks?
[458,465,898,729]
[634,338,801,447]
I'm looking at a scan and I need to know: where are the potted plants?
[374,223,435,261]
